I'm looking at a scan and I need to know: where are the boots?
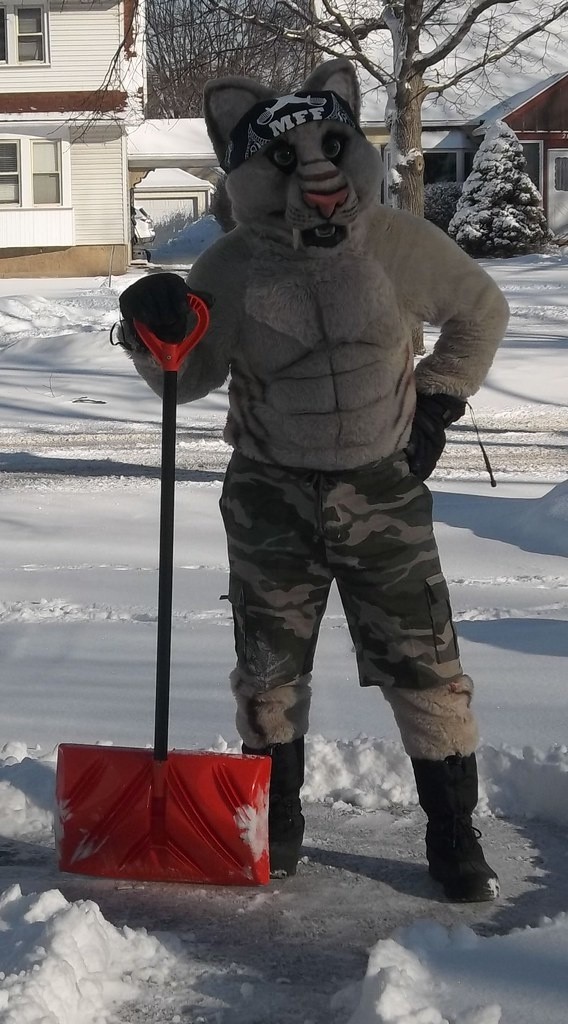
[242,736,305,879]
[411,752,500,902]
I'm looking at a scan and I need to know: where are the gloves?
[403,389,466,481]
[119,273,216,353]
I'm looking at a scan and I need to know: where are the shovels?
[56,293,273,889]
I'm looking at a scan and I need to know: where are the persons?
[119,58,511,903]
